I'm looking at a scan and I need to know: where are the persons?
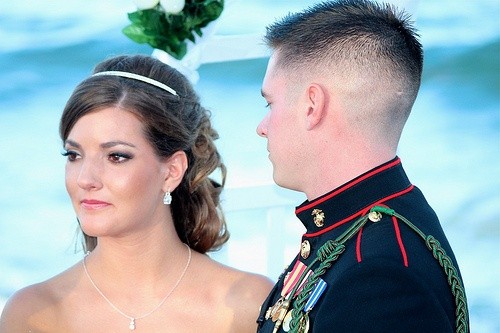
[0,55,276,333]
[255,0,470,333]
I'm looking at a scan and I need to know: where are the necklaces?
[82,243,191,330]
